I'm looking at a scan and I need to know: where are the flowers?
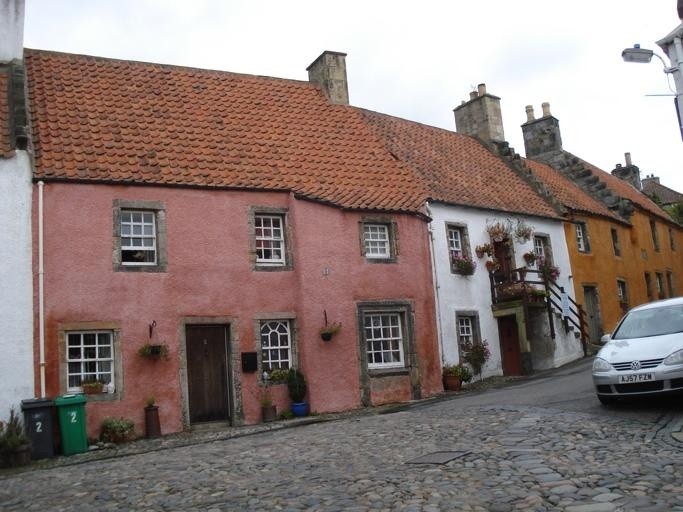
[454,254,477,270]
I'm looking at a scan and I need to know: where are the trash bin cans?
[20,394,89,461]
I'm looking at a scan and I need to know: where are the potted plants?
[245,368,310,422]
[100,395,162,443]
[442,365,472,391]
[81,381,103,393]
[138,342,170,359]
[319,325,343,340]
[478,215,561,303]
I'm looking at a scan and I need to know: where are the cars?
[592,297,683,403]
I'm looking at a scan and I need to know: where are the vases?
[461,269,469,274]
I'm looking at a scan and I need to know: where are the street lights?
[621,38,683,80]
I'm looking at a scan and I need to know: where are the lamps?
[619,46,681,96]
